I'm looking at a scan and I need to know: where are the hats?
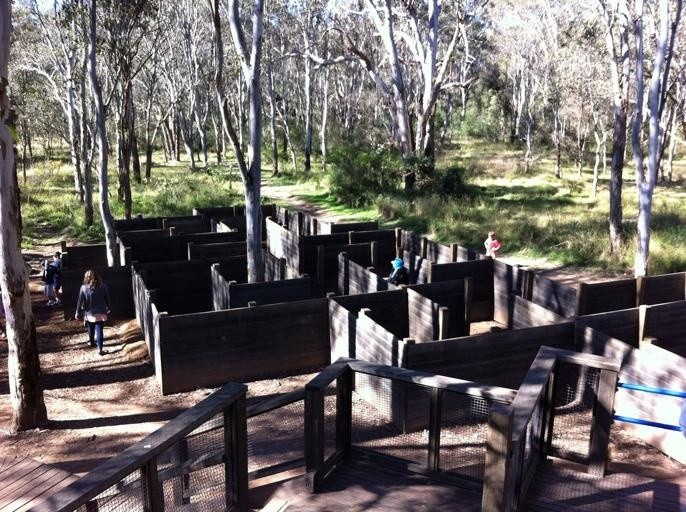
[488,231,496,236]
[391,259,395,265]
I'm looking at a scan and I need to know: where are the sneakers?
[46,299,61,306]
[87,342,108,355]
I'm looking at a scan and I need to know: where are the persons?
[484,232,503,260]
[41,260,56,306]
[381,257,409,286]
[75,270,111,356]
[50,251,63,305]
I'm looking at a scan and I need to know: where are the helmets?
[396,258,404,266]
